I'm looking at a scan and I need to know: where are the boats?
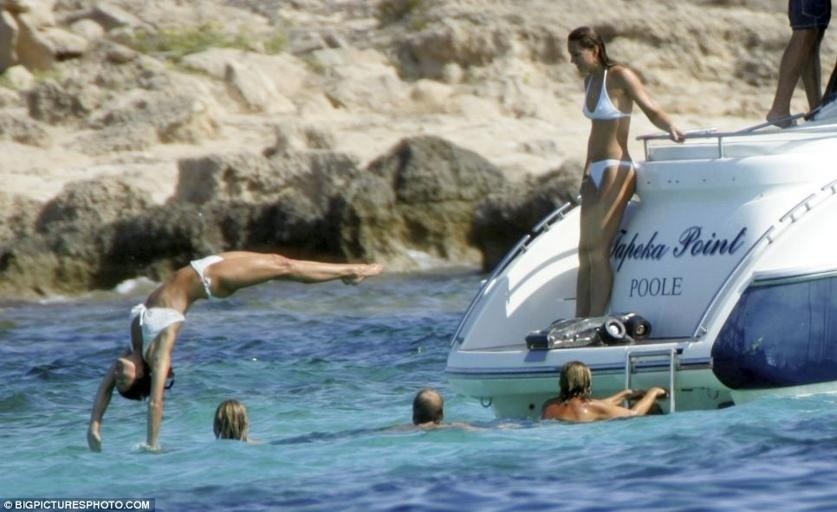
[444,98,837,421]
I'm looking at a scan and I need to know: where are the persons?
[87,250,386,455]
[766,0,832,130]
[540,360,667,424]
[567,25,684,316]
[212,399,262,446]
[387,389,459,433]
